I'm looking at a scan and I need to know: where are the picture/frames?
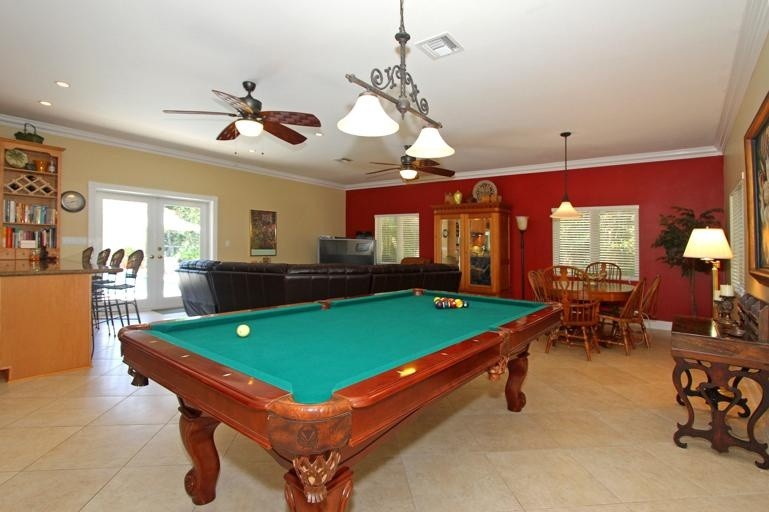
[744,93,769,281]
[249,209,277,256]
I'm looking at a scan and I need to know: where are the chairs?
[81,246,144,338]
[527,262,661,360]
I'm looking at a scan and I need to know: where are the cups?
[26,163,35,170]
[34,159,48,172]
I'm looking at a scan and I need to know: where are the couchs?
[174,259,461,317]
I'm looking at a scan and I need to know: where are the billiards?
[434,297,468,308]
[238,324,250,336]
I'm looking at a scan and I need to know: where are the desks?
[118,288,564,512]
[670,293,769,470]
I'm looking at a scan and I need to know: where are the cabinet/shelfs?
[0,136,66,260]
[430,203,512,297]
[0,259,30,271]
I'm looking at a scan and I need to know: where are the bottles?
[40,246,48,259]
[30,262,40,272]
[46,154,56,173]
[39,260,49,272]
[29,251,39,261]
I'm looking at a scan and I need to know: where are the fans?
[163,81,321,145]
[365,145,454,177]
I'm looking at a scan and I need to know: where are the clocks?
[61,190,85,213]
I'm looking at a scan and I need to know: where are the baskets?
[14,123,44,143]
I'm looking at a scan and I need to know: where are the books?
[1,199,56,249]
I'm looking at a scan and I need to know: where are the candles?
[719,284,736,297]
[713,290,723,301]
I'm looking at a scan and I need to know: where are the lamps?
[337,0,455,159]
[399,170,417,180]
[681,226,736,326]
[514,215,529,300]
[549,132,581,220]
[234,117,263,137]
